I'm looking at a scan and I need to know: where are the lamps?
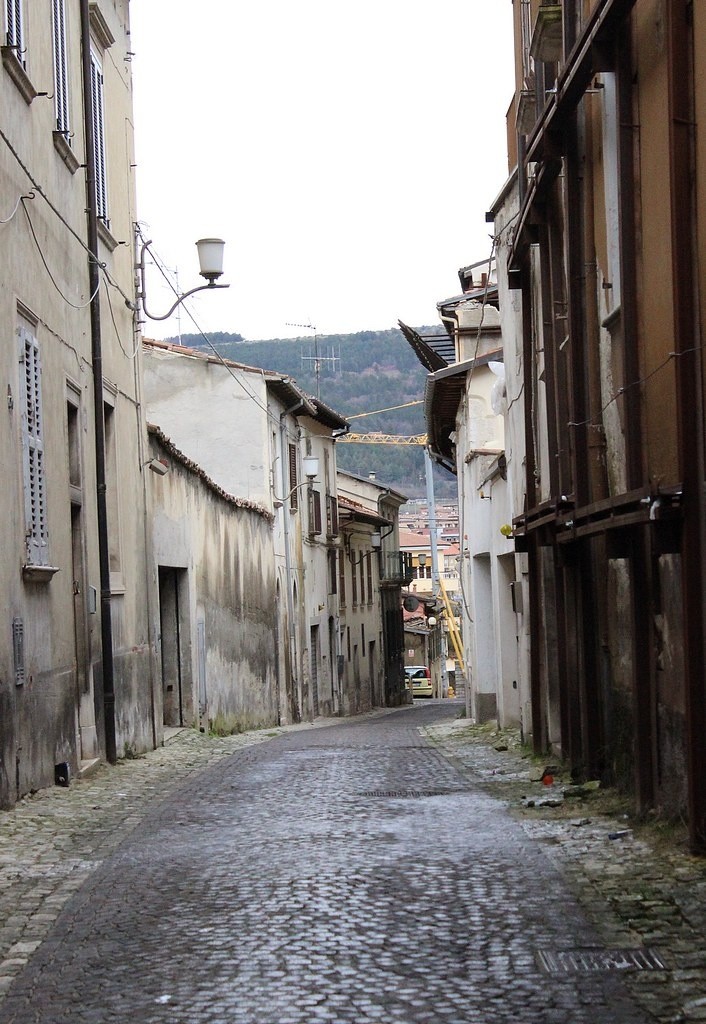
[428,617,436,629]
[270,455,321,501]
[405,554,426,577]
[500,525,514,539]
[141,238,230,320]
[480,491,491,500]
[343,532,381,565]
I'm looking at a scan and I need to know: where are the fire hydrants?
[448,687,454,698]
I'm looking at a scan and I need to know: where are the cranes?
[332,399,428,446]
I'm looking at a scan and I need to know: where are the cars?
[404,665,437,698]
